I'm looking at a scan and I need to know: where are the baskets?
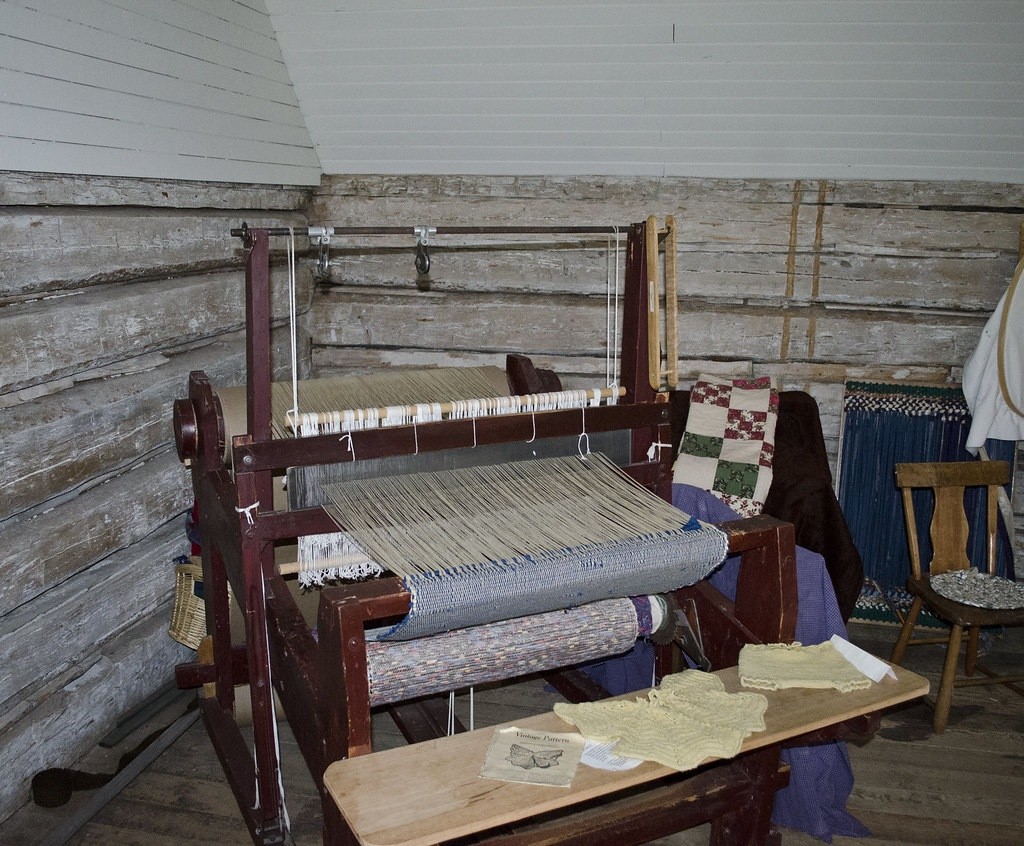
[167,564,231,651]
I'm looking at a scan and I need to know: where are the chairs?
[890,459,1024,736]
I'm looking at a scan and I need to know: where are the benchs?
[319,632,932,846]
